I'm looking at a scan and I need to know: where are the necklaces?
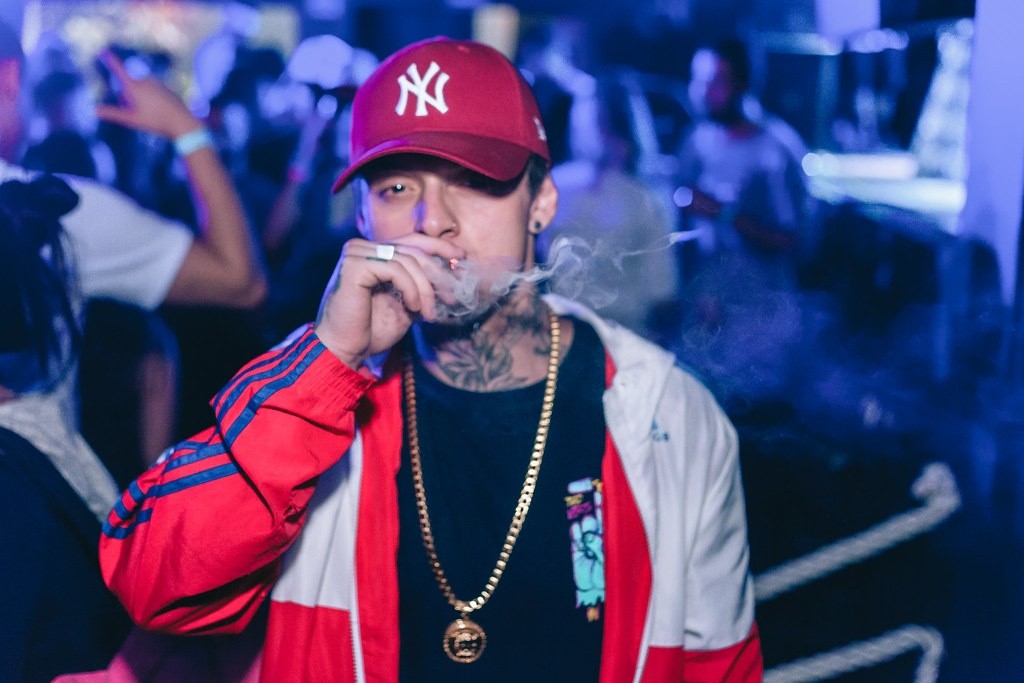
[403,309,559,664]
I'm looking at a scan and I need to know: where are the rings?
[375,245,395,260]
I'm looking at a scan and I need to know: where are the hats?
[331,36,551,195]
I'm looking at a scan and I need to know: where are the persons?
[99,38,766,683]
[513,20,1011,683]
[0,23,382,683]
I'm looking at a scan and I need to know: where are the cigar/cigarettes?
[439,256,458,269]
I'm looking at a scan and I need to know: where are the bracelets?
[173,127,216,158]
